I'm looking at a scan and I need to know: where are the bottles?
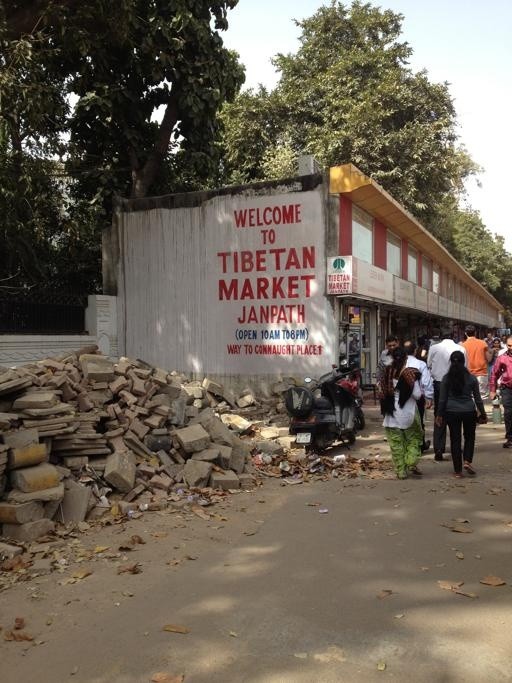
[491,404,502,424]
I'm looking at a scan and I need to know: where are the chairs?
[359,373,377,406]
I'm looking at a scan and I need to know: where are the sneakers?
[407,464,424,478]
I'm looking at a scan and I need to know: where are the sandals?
[462,460,476,475]
[452,469,462,478]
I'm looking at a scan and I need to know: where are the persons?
[376,325,512,478]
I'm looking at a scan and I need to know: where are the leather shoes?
[433,449,446,462]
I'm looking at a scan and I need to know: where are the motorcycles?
[285,359,366,457]
